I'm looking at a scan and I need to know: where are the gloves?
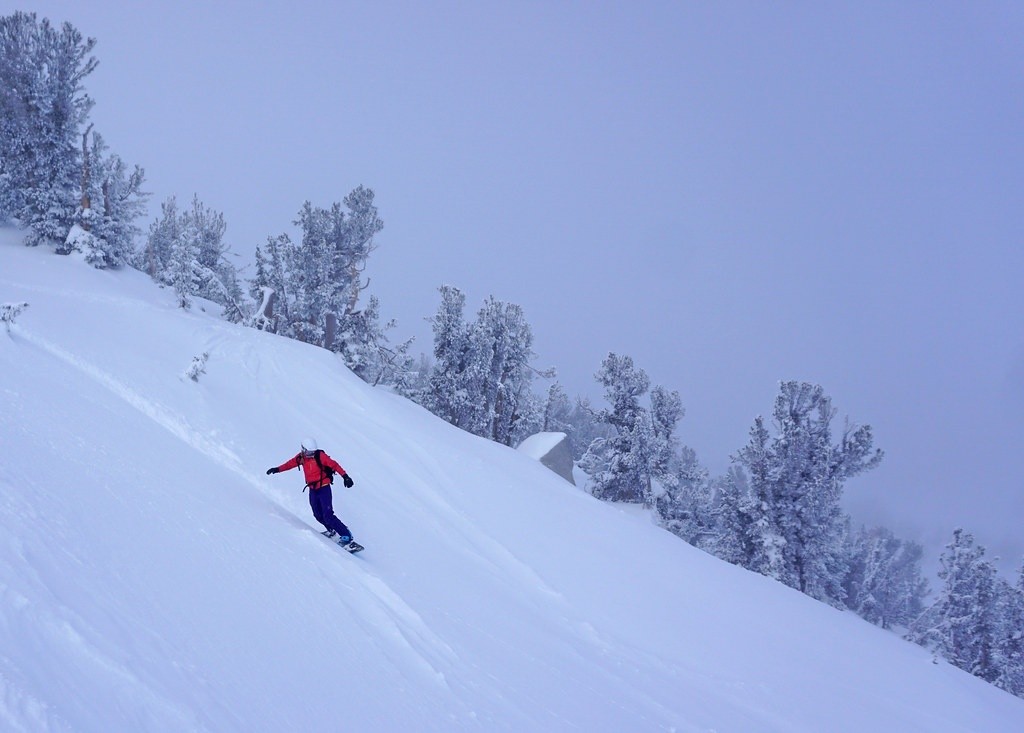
[266,468,277,474]
[343,475,354,488]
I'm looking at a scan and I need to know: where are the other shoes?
[322,530,336,538]
[337,534,353,547]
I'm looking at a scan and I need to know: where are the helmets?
[301,438,318,452]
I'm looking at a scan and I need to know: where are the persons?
[266,437,354,547]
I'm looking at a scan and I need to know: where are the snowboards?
[321,531,365,554]
